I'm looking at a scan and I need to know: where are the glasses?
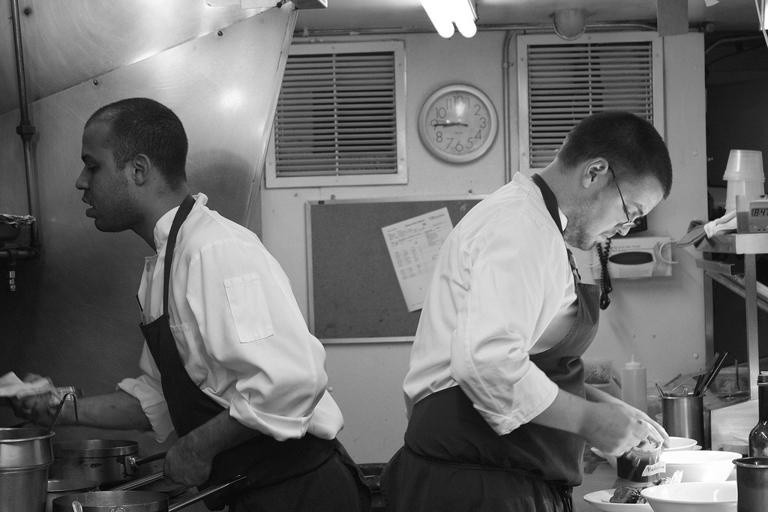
[608,166,637,230]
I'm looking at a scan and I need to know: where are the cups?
[731,455,767,511]
[662,392,704,443]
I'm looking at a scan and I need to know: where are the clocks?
[416,82,499,164]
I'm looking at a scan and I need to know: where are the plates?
[583,487,652,511]
[663,450,741,481]
[590,436,695,458]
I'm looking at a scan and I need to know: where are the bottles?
[749,411,768,458]
[621,354,648,415]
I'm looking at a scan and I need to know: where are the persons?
[10,96,373,511]
[373,110,672,511]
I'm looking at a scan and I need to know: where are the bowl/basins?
[640,481,739,511]
[721,150,766,182]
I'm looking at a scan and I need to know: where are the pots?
[1,426,59,511]
[1,377,82,447]
[52,474,247,512]
[49,438,169,492]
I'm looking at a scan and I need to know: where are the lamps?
[419,0,478,39]
[552,8,587,42]
